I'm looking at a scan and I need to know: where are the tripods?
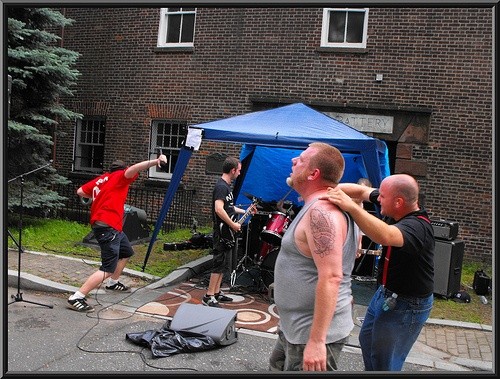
[235,217,258,282]
[7,164,53,308]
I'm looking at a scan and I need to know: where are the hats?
[452,291,471,303]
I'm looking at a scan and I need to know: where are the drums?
[260,248,281,292]
[258,241,274,261]
[260,210,292,248]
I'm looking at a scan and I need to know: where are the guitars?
[220,198,262,247]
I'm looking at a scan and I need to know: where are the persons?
[318,173,440,372]
[68,154,167,313]
[201,156,257,307]
[268,142,359,372]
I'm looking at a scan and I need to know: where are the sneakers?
[66,297,95,312]
[202,295,224,308]
[106,281,131,292]
[215,291,233,303]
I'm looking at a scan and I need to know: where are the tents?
[140,104,390,272]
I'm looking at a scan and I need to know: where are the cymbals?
[243,192,275,210]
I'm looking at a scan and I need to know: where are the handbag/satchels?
[473,269,491,296]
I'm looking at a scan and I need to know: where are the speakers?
[170,302,239,346]
[432,239,465,297]
